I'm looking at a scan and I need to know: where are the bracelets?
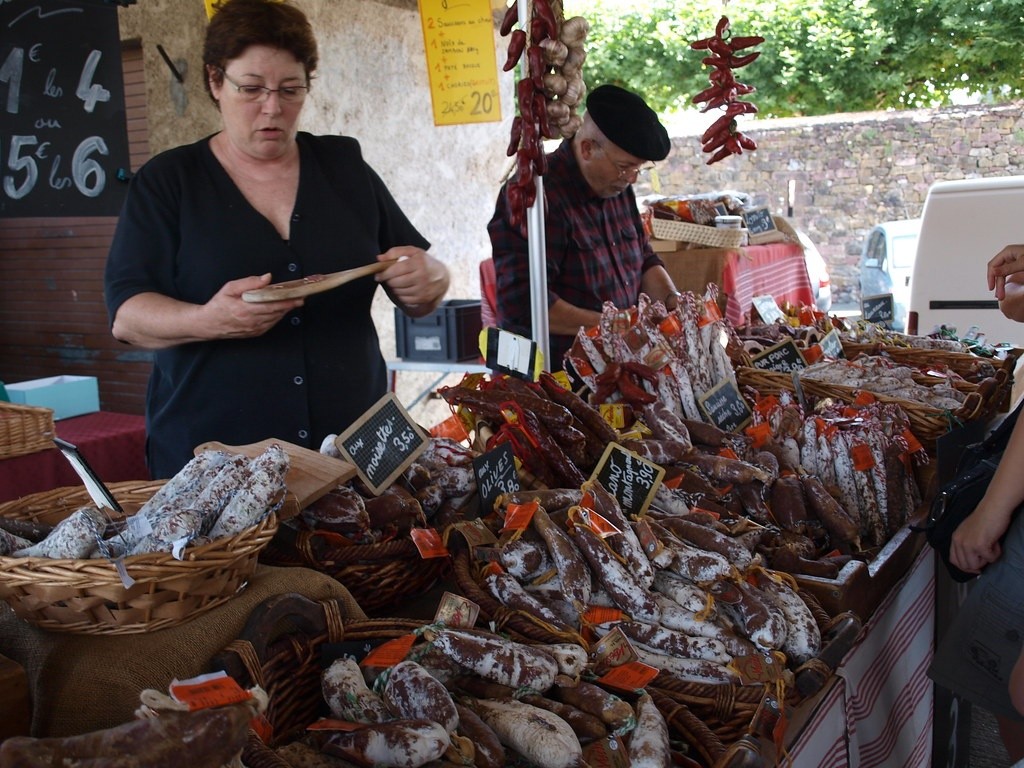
[664,290,681,310]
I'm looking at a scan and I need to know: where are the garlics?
[539,16,588,137]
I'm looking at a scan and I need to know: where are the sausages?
[0,278,938,768]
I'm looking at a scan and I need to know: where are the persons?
[103,0,452,482]
[924,244,1024,767]
[485,85,681,374]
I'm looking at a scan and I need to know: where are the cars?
[853,218,921,334]
[793,229,832,318]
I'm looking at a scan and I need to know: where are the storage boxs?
[392,298,481,364]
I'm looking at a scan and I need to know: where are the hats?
[586,85,671,162]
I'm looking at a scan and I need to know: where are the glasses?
[218,67,310,101]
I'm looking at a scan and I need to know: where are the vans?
[908,173,1024,349]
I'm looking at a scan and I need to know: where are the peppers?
[500,0,559,239]
[691,16,765,166]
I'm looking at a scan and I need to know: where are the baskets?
[738,300,1009,445]
[0,480,282,636]
[0,401,56,459]
[242,487,863,768]
